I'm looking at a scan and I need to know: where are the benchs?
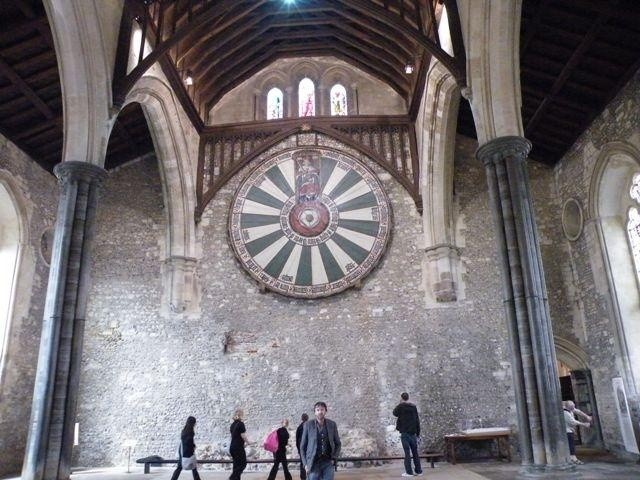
[135,453,444,473]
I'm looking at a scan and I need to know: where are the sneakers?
[401,472,424,476]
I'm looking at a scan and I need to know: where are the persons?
[226,407,256,480]
[266,418,294,480]
[171,416,201,480]
[300,402,342,480]
[563,399,594,465]
[392,391,424,477]
[294,413,309,480]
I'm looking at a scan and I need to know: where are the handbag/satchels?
[179,440,197,470]
[262,429,279,455]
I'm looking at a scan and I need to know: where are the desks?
[444,430,511,465]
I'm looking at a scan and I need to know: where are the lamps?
[184,69,195,86]
[403,62,417,76]
